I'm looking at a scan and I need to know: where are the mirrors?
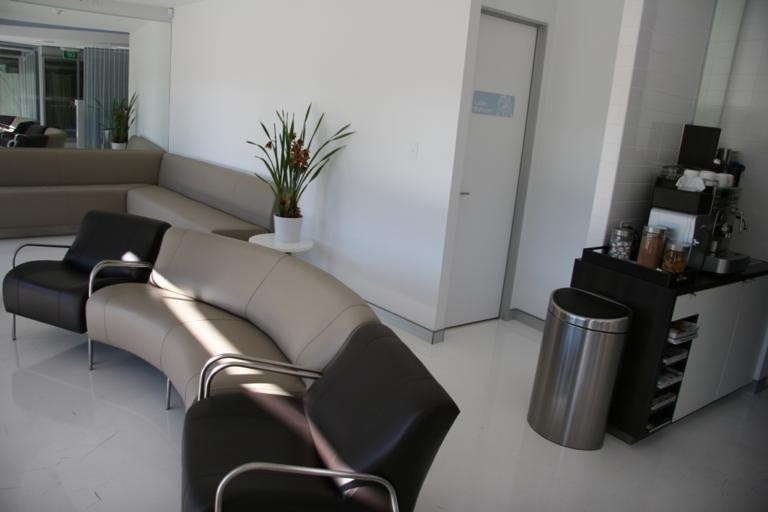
[0,16,132,148]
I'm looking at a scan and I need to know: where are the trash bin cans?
[527,287,633,450]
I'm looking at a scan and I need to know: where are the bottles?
[662,241,690,274]
[608,228,633,261]
[636,224,668,268]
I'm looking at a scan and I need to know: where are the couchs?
[0,146,163,241]
[127,151,284,243]
[83,223,380,414]
[0,113,75,148]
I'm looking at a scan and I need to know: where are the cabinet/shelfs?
[570,259,700,447]
[672,276,768,423]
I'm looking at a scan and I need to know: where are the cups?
[683,169,734,188]
[661,165,679,179]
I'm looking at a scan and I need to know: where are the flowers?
[244,102,356,215]
[90,91,138,141]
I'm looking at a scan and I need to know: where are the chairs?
[3,207,172,369]
[179,318,464,511]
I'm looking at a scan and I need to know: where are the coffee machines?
[646,179,751,273]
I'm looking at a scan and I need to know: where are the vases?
[111,142,128,150]
[272,211,304,243]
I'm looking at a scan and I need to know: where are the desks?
[248,232,316,255]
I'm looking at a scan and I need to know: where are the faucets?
[732,208,749,233]
